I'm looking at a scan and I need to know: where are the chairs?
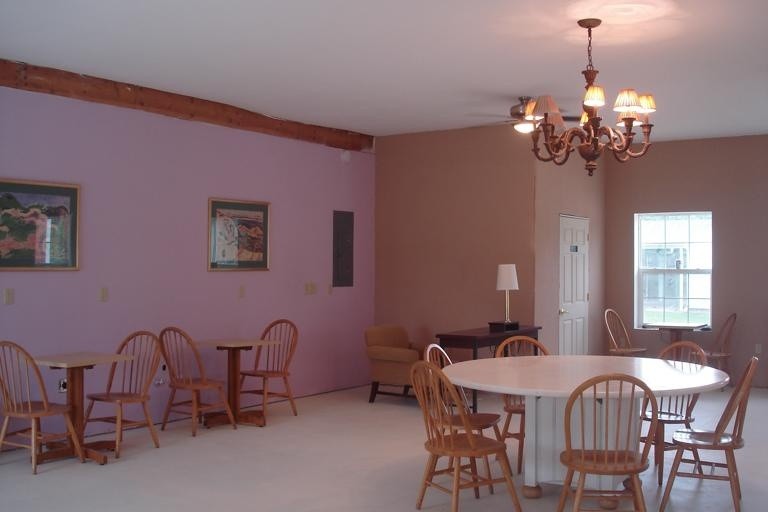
[424,340,500,495]
[660,356,756,508]
[160,327,237,437]
[411,360,522,510]
[690,312,739,391]
[364,324,426,402]
[559,373,660,512]
[604,308,645,358]
[0,341,85,475]
[82,331,159,458]
[494,334,555,477]
[639,341,709,480]
[240,320,314,416]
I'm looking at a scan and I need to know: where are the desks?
[192,340,282,429]
[641,321,709,362]
[441,356,732,510]
[25,353,136,466]
[435,324,544,413]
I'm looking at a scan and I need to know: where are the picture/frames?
[207,198,271,271]
[0,179,80,271]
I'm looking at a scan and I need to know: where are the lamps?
[489,264,519,332]
[514,120,537,134]
[532,19,655,176]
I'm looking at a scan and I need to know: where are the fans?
[464,96,581,121]
